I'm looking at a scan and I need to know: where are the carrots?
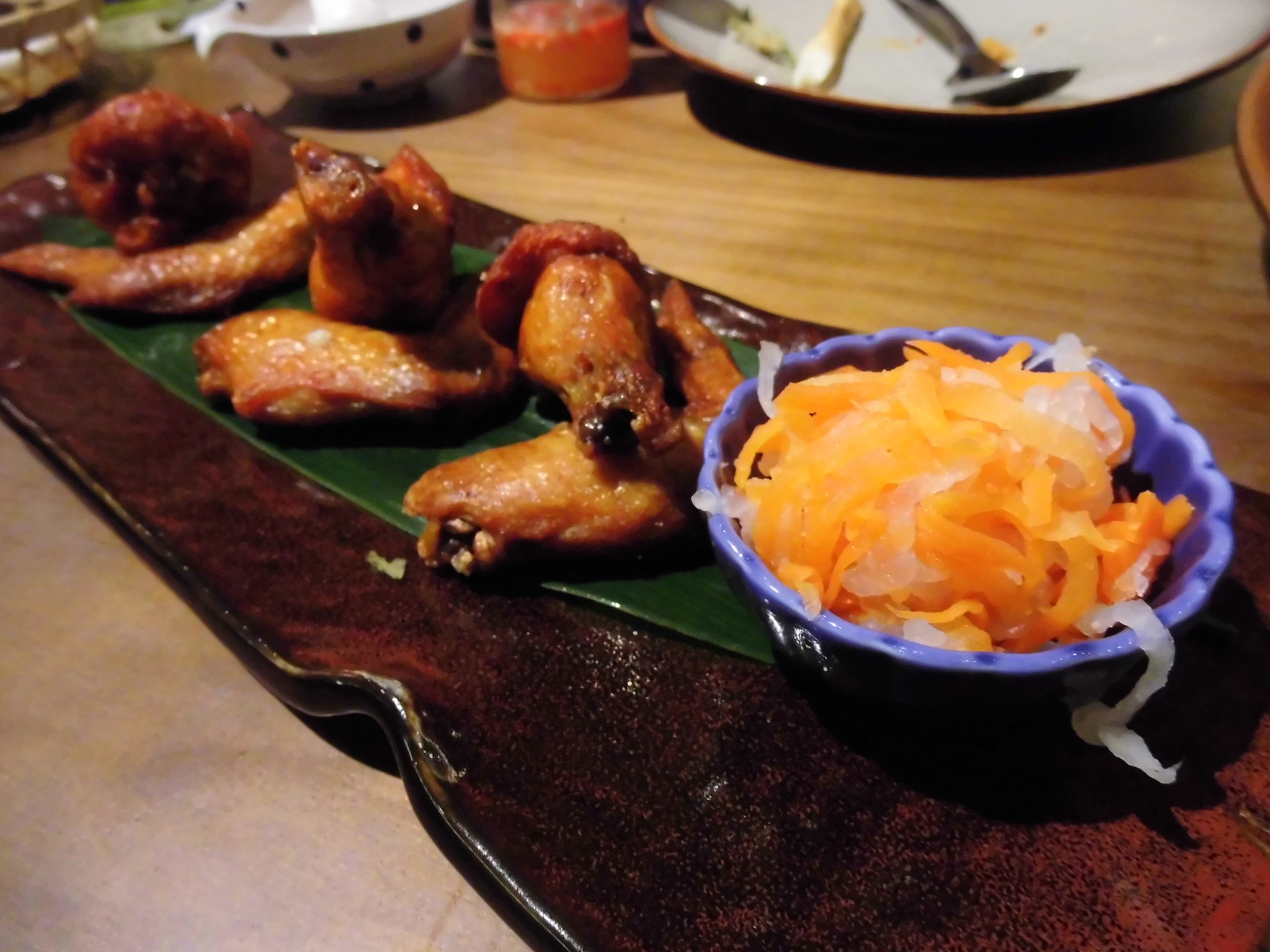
[735,336,1190,653]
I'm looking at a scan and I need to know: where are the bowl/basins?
[179,0,478,110]
[696,325,1235,750]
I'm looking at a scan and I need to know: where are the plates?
[3,129,1269,952]
[639,0,1268,146]
[1234,58,1270,214]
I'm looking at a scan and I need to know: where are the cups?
[487,0,633,106]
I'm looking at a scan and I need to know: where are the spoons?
[890,1,1079,109]
